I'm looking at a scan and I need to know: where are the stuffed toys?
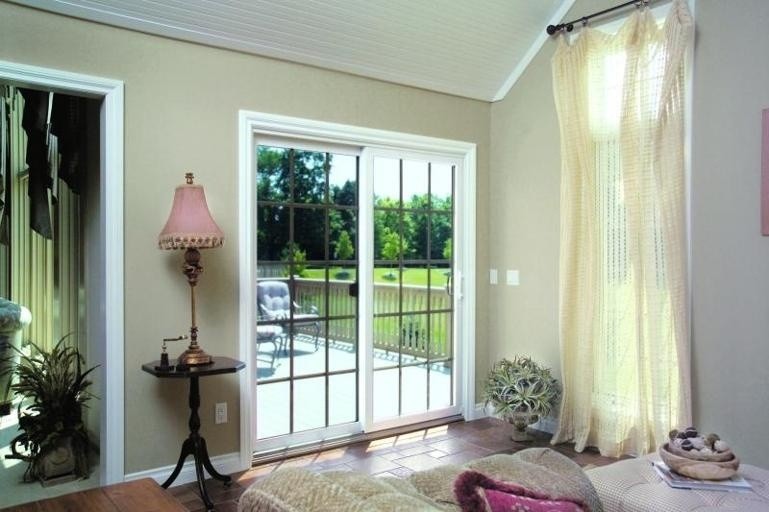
[665,425,731,458]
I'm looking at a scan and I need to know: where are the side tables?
[142,356,246,509]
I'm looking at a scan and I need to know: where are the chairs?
[257,281,321,356]
[257,314,284,373]
[0,297,33,416]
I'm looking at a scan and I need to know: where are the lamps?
[156,171,226,367]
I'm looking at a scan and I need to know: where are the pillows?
[451,471,591,511]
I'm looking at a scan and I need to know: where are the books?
[652,461,751,491]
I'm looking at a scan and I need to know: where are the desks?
[583,450,769,512]
[0,478,194,512]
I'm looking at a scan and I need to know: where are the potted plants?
[483,354,561,442]
[0,331,102,488]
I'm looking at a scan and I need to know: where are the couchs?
[236,445,599,512]
